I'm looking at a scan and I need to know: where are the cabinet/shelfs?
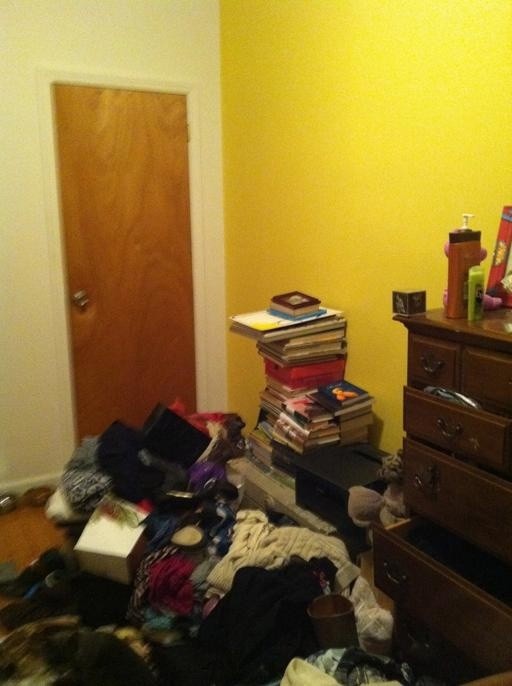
[371,308,512,686]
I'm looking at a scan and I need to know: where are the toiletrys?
[469,266,484,324]
[467,266,485,322]
[449,228,480,321]
[446,212,482,319]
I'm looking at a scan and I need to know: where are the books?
[228,310,374,479]
[270,291,322,316]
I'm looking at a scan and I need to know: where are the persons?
[0,618,156,686]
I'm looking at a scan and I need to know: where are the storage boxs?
[224,458,336,537]
